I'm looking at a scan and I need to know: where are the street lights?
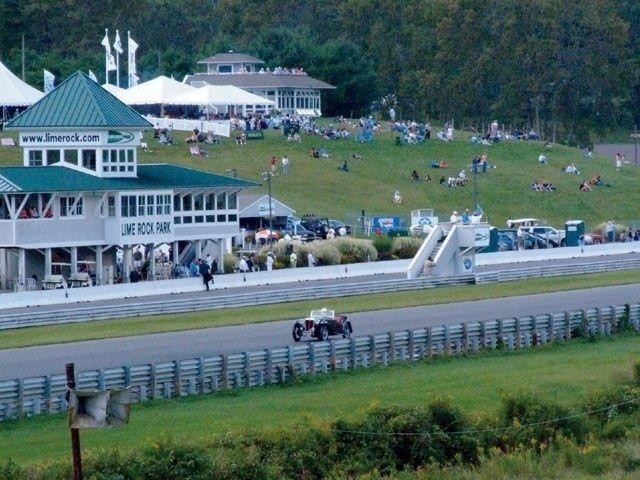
[260,170,280,247]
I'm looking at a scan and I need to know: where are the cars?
[289,224,315,243]
[586,233,606,244]
[327,220,351,237]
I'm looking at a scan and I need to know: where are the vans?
[498,227,545,249]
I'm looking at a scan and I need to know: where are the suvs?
[305,219,329,240]
[520,226,567,246]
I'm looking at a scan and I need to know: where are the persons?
[308,251,317,267]
[532,146,627,193]
[243,222,348,247]
[517,226,524,248]
[265,252,274,270]
[578,229,640,246]
[140,111,454,160]
[342,160,349,171]
[395,190,402,204]
[270,154,289,175]
[237,66,304,76]
[411,155,490,187]
[290,251,298,268]
[471,120,539,145]
[450,209,484,225]
[142,252,257,290]
[0,205,53,219]
